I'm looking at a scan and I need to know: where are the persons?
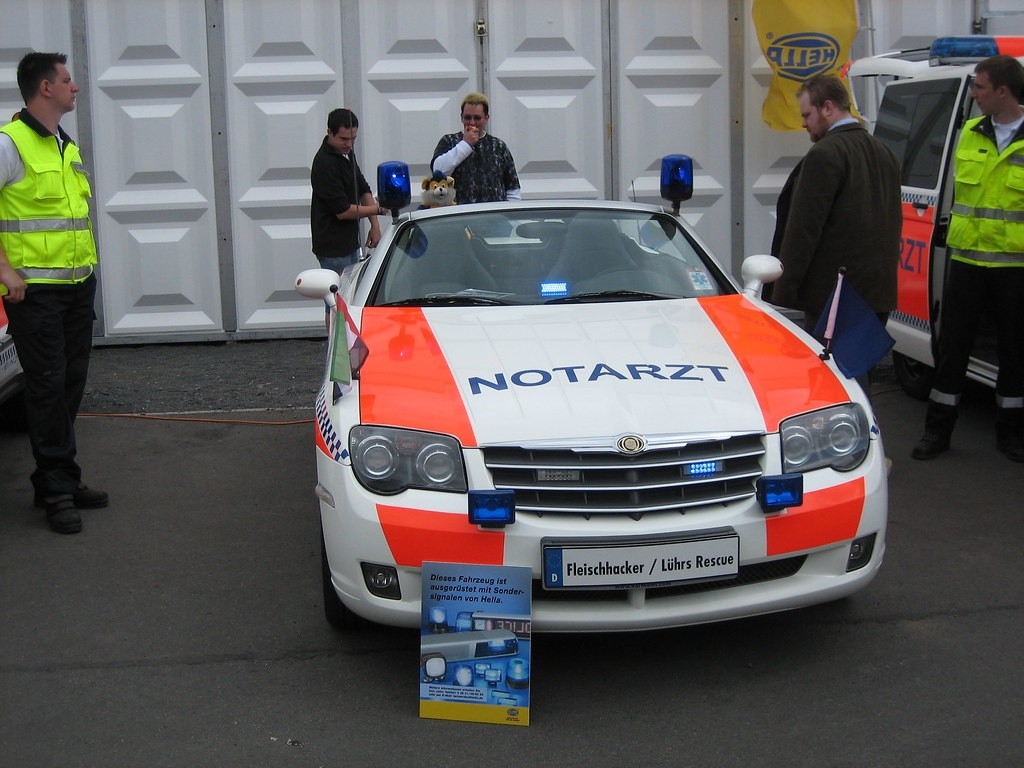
[0,51,109,535]
[761,71,900,400]
[912,55,1024,463]
[310,107,383,336]
[431,93,521,206]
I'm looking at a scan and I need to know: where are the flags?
[327,294,369,407]
[811,273,897,378]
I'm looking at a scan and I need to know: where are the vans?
[848,36,1024,408]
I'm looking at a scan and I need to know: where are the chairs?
[397,223,500,294]
[545,214,633,288]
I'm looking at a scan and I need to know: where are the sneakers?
[32,487,109,509]
[49,500,83,534]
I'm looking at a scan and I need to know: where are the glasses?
[462,114,486,121]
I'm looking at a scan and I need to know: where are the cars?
[294,155,889,639]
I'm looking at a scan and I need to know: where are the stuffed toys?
[416,169,456,210]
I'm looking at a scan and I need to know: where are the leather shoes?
[911,432,951,460]
[994,438,1024,462]
